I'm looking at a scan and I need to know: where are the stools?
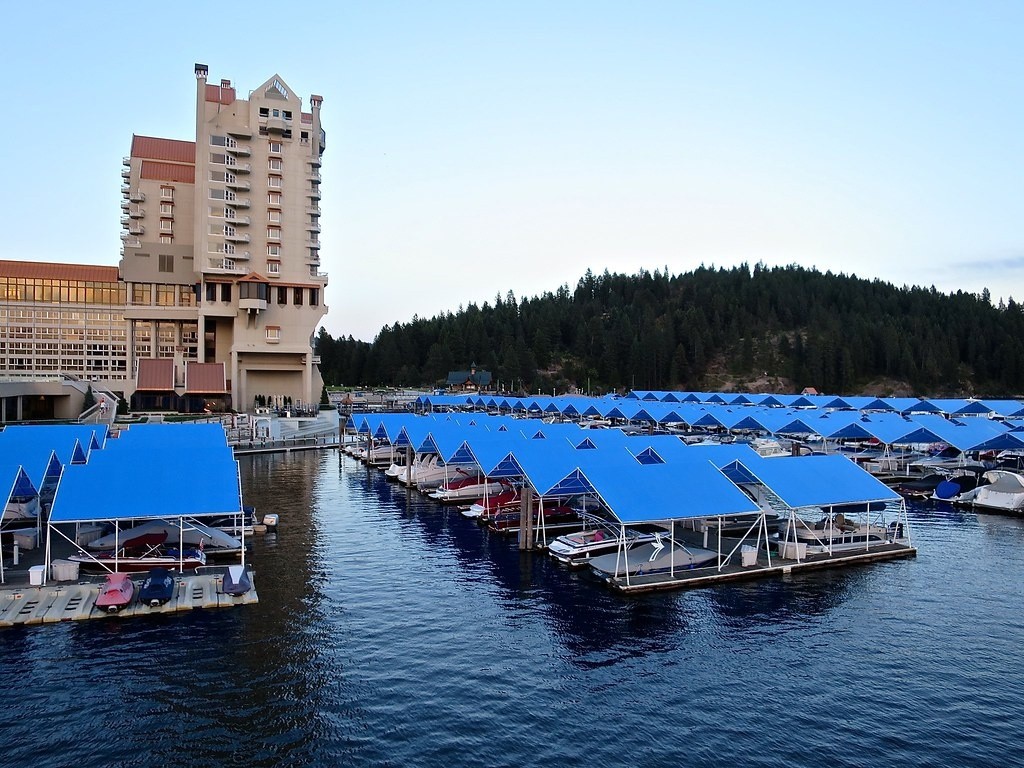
[28,564,45,585]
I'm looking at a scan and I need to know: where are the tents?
[0,422,245,585]
[347,388,1024,590]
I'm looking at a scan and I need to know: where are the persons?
[99,397,108,414]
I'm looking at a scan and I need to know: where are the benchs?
[12,532,37,550]
[577,497,599,507]
[51,559,79,581]
[77,525,102,546]
[778,541,807,560]
[740,545,758,568]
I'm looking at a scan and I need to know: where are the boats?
[930,465,992,504]
[138,570,175,606]
[67,544,207,576]
[95,573,134,613]
[222,564,252,597]
[98,389,1023,571]
[778,502,888,554]
[587,539,719,575]
[546,508,674,565]
[971,470,1024,511]
